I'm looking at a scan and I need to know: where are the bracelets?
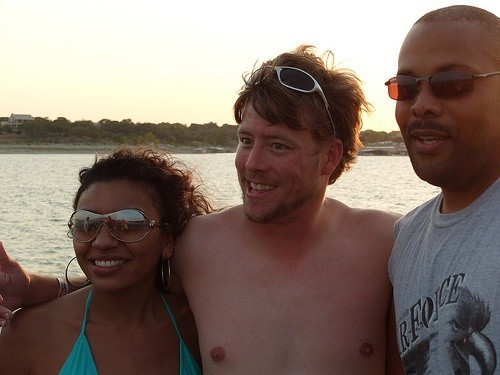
[56,277,68,297]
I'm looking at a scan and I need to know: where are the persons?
[388,4,500,374]
[1,149,204,375]
[84,215,130,234]
[0,44,405,374]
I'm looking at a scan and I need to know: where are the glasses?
[245,66,340,136]
[68,210,164,243]
[384,71,500,100]
